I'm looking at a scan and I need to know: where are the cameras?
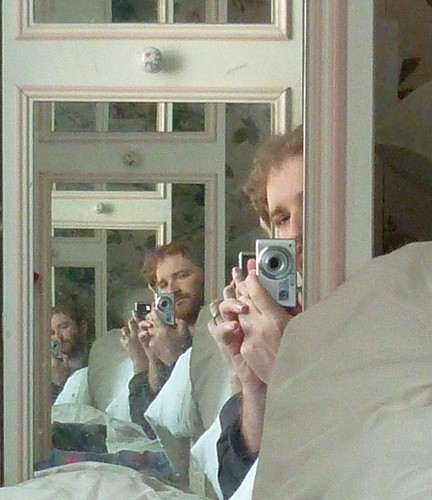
[51,339,62,359]
[239,252,256,279]
[257,238,298,307]
[136,302,152,321]
[155,291,177,326]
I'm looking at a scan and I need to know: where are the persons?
[184,123,308,500]
[48,301,97,406]
[119,238,202,444]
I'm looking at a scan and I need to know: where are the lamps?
[374,73,432,198]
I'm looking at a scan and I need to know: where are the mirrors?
[13,85,305,500]
[13,0,293,42]
